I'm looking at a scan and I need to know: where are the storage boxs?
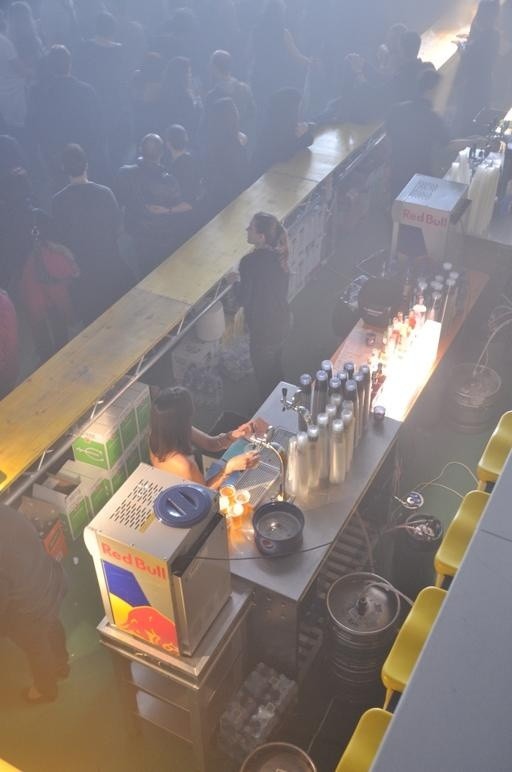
[123,445,141,478]
[57,458,110,519]
[102,464,126,499]
[99,401,140,451]
[30,473,90,544]
[15,497,66,562]
[138,433,154,466]
[118,381,154,436]
[71,421,123,472]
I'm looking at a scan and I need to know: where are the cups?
[219,484,250,530]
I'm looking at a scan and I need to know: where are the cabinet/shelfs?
[97,591,257,771]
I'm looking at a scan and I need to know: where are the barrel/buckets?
[239,743,317,772]
[443,361,502,437]
[326,572,401,700]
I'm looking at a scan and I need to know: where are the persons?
[150,388,268,493]
[0,471,71,700]
[1,0,488,397]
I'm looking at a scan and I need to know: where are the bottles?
[33,510,57,538]
[216,662,297,753]
[184,363,224,405]
[383,262,471,359]
[229,341,252,369]
[289,360,386,496]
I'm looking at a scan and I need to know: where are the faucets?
[279,387,312,427]
[243,425,286,501]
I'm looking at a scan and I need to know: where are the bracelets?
[216,433,228,450]
[226,431,236,441]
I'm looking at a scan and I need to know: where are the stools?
[432,488,492,589]
[475,410,512,491]
[381,585,448,711]
[333,708,393,772]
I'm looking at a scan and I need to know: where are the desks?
[371,531,512,772]
[442,144,511,249]
[478,450,512,541]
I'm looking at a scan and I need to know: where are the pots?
[253,501,304,557]
